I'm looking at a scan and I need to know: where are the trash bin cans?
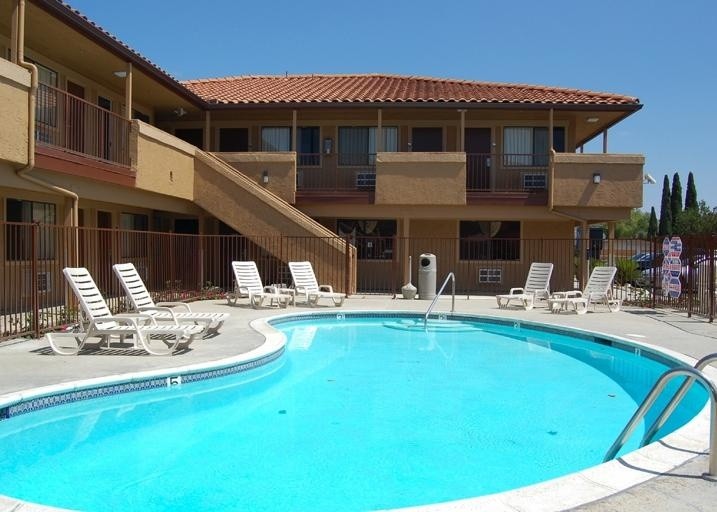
[419,253,436,300]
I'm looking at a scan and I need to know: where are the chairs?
[276,261,347,307]
[547,267,623,315]
[525,337,552,353]
[110,262,229,338]
[45,266,206,356]
[288,325,319,350]
[496,262,562,311]
[224,261,291,310]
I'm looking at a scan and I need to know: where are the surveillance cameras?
[644,174,656,185]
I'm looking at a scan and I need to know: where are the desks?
[120,316,152,348]
[567,290,586,308]
[551,291,576,311]
[270,288,296,309]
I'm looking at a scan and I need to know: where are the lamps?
[262,171,270,184]
[593,172,601,185]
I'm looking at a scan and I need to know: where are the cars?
[640,254,716,294]
[625,252,664,271]
